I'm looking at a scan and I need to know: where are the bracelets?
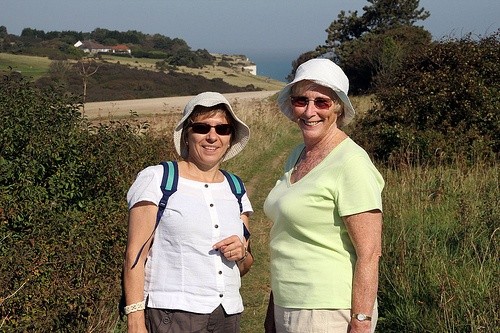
[123,300,145,314]
[239,246,248,263]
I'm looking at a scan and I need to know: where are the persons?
[122,91,255,333]
[263,58,385,333]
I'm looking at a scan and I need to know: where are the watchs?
[351,313,372,321]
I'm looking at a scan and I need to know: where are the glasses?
[189,117,234,135]
[289,94,338,110]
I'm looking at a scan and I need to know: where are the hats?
[174,92,250,162]
[278,58,356,130]
